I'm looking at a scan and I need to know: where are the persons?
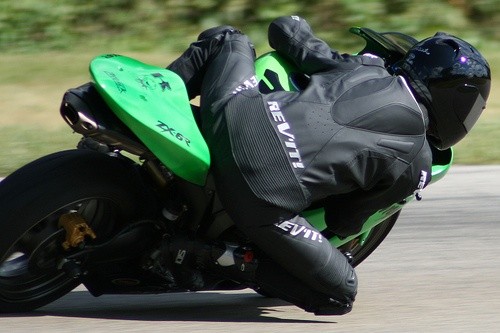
[77,16,491,315]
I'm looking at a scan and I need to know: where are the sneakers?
[210,240,259,281]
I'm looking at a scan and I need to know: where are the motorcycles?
[0,24,454,317]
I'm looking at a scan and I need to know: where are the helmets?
[401,32,491,150]
